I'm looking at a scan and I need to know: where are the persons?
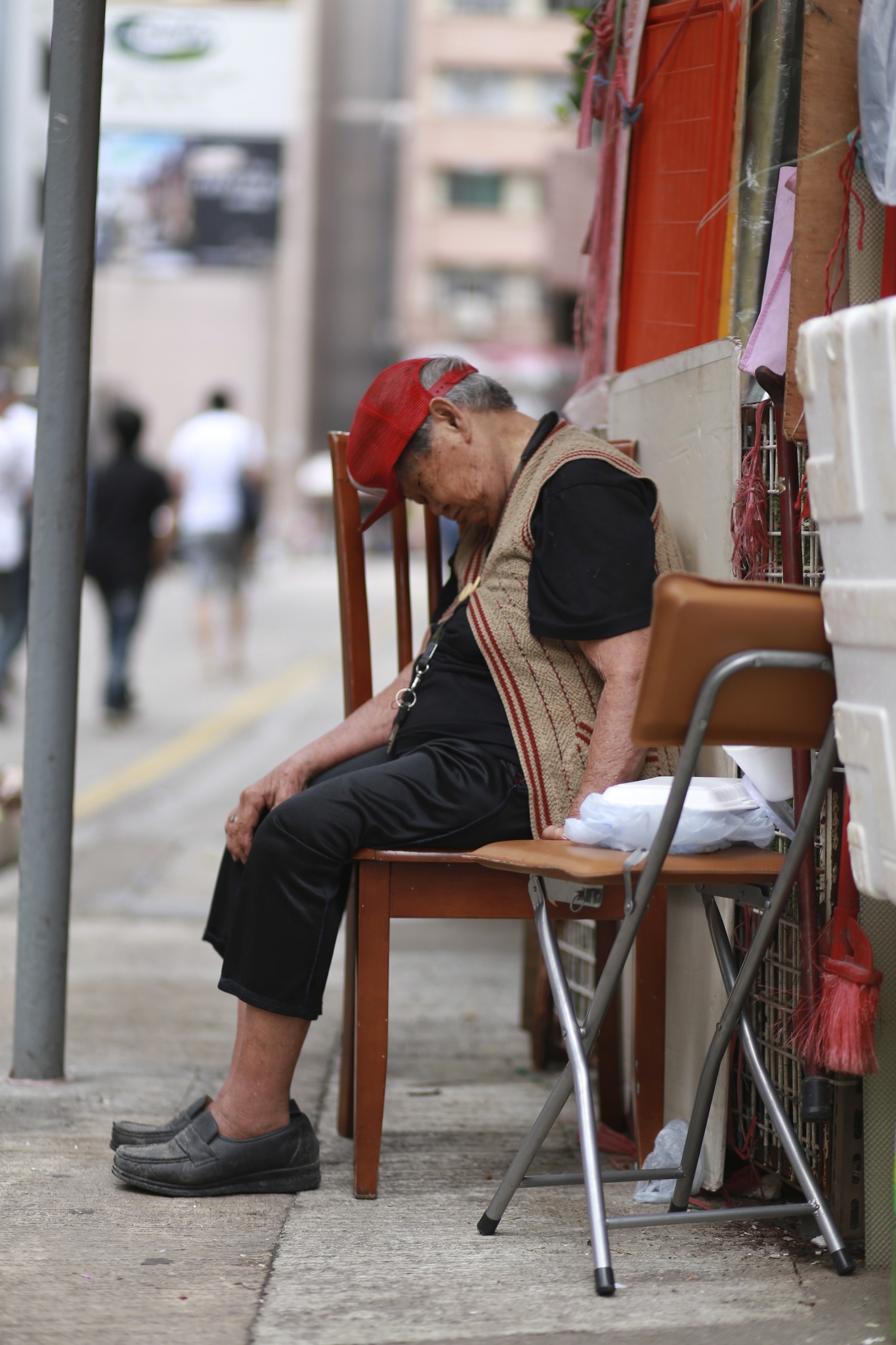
[0,376,39,726]
[83,402,177,710]
[106,357,698,1196]
[164,380,270,679]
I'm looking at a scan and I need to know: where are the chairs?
[321,427,669,1204]
[467,561,856,1305]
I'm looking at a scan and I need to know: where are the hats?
[343,357,479,534]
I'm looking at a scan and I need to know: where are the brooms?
[773,775,887,1084]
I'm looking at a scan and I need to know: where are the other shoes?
[103,684,134,720]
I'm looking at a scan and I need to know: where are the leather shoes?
[112,1094,300,1154]
[111,1101,323,1196]
